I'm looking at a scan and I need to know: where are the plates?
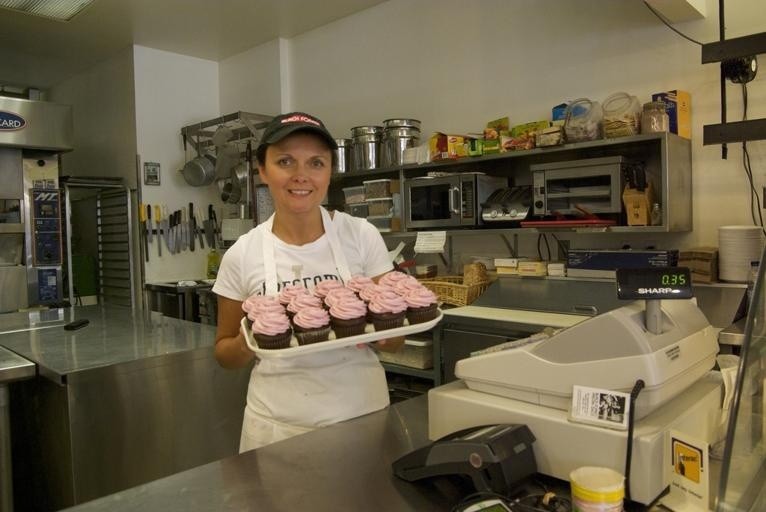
[718,225,766,283]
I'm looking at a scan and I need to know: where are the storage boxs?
[342,179,401,234]
[376,335,434,370]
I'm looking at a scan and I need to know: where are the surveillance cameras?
[722,55,758,84]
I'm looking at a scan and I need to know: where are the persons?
[211,111,395,456]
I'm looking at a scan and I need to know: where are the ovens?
[530,155,630,219]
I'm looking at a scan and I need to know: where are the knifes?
[627,163,647,193]
[141,203,220,261]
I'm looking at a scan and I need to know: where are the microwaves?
[404,173,509,230]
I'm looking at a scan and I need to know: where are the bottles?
[207,249,221,279]
[564,92,669,144]
[748,260,760,310]
[650,204,662,225]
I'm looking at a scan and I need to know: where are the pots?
[334,118,421,174]
[181,131,248,204]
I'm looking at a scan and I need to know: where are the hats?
[258,112,338,150]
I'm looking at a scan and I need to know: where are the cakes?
[241,270,437,349]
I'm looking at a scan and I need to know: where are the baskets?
[415,276,498,307]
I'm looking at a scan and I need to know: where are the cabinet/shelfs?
[330,132,691,236]
[379,323,442,404]
